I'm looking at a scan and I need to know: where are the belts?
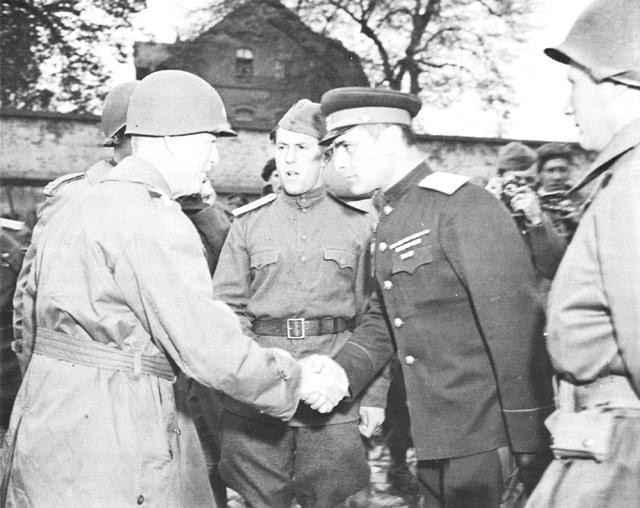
[252,316,349,342]
[30,327,177,384]
[552,374,640,418]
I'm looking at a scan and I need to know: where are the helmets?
[99,77,142,139]
[125,67,240,141]
[539,0,640,90]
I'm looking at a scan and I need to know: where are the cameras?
[497,177,523,217]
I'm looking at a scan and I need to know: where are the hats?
[495,142,539,171]
[316,84,425,148]
[277,97,328,141]
[533,141,575,162]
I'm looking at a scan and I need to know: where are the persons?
[0,68,587,508]
[527,0,640,508]
